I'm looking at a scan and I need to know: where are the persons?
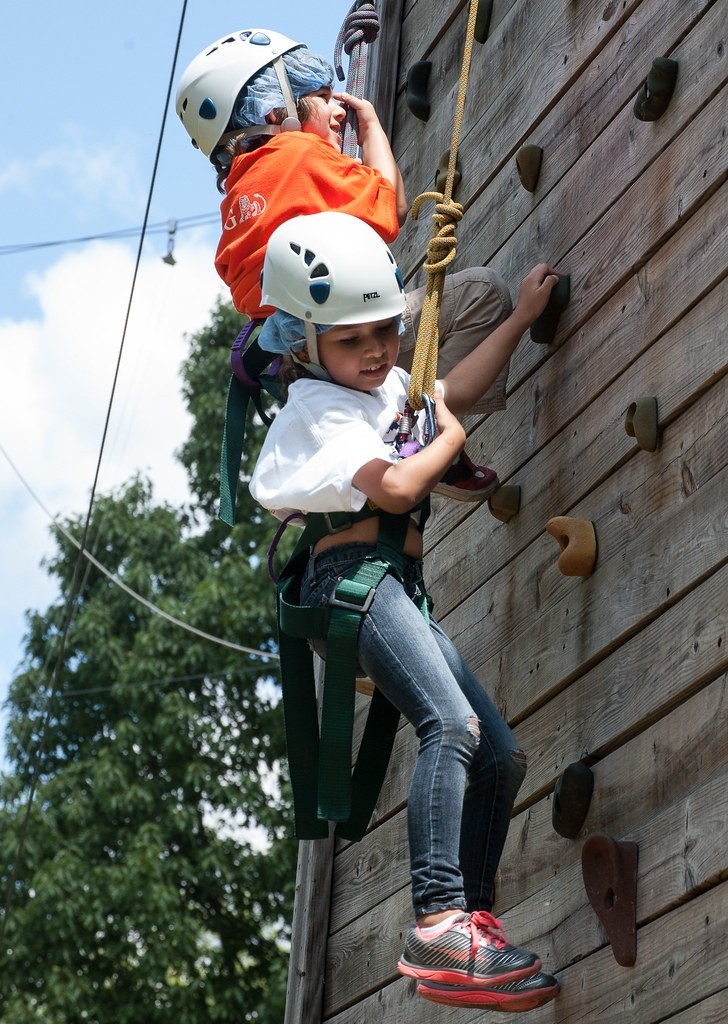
[251,213,560,1010]
[174,30,512,505]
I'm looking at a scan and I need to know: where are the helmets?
[259,211,407,326]
[175,27,309,166]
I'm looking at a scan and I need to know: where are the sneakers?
[396,910,542,986]
[417,971,561,1012]
[431,449,500,503]
